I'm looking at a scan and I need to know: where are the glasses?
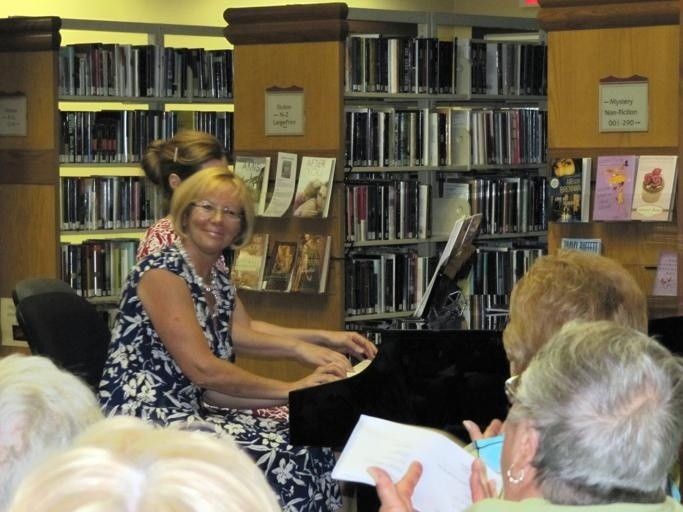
[504,374,533,417]
[190,200,244,220]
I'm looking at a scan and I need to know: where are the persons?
[95,164,357,512]
[1,352,104,512]
[134,127,379,422]
[462,248,650,445]
[11,411,290,512]
[365,317,681,512]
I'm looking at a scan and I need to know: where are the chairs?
[11,275,79,301]
[14,291,111,385]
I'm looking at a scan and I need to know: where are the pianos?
[289,330,510,511]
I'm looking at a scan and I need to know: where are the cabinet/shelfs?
[222,3,547,380]
[534,0,682,329]
[0,17,233,362]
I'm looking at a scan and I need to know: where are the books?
[56,43,232,298]
[230,152,337,293]
[549,155,680,299]
[346,34,549,369]
